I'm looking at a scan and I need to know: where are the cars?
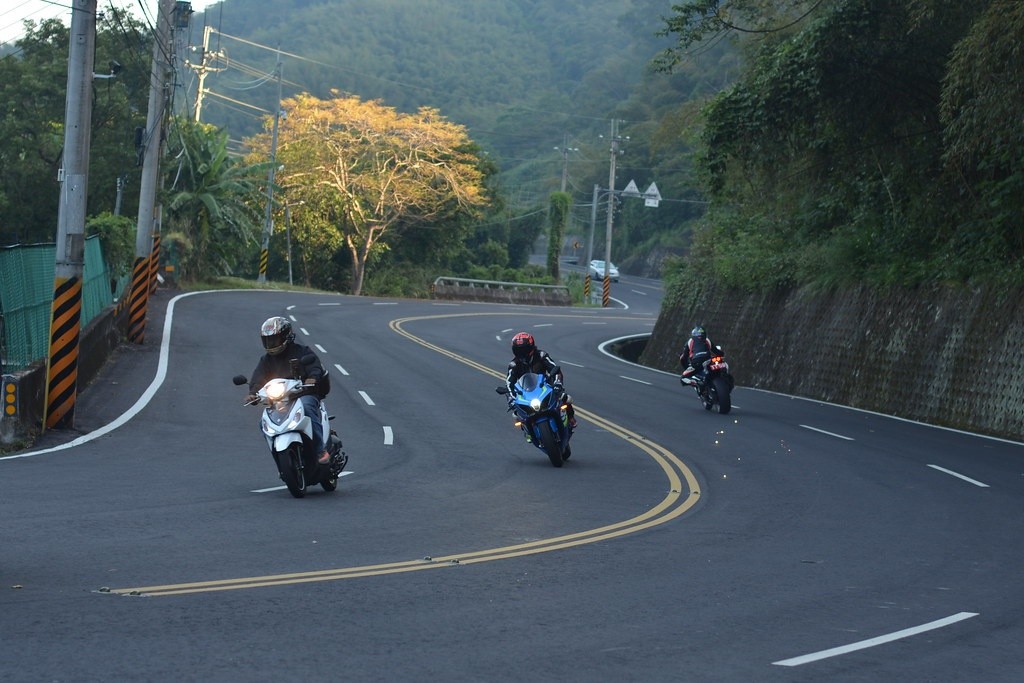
[589,260,620,282]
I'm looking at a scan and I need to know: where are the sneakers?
[318,448,329,465]
[568,417,577,428]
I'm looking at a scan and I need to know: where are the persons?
[506,332,577,442]
[245,317,331,464]
[680,326,724,395]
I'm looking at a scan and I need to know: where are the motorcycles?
[232,353,348,499]
[680,346,731,414]
[495,366,574,466]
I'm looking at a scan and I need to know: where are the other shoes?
[696,381,703,395]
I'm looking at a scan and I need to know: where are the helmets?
[691,326,706,337]
[260,316,295,356]
[512,332,536,358]
